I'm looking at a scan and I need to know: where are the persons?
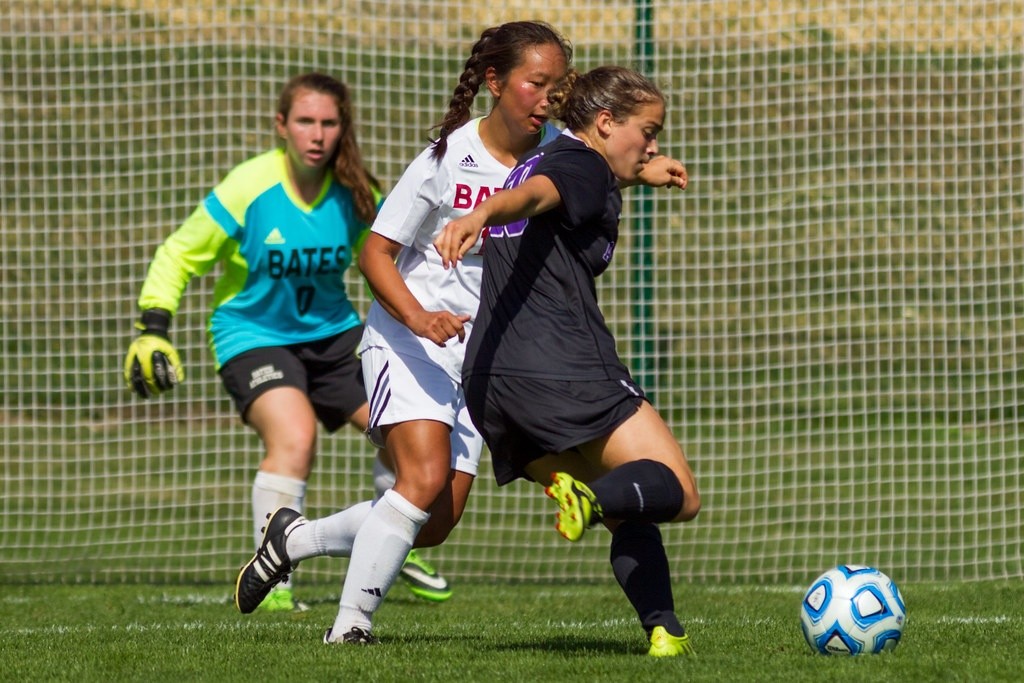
[123,68,458,614]
[232,20,690,650]
[433,66,704,663]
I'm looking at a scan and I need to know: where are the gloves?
[123,307,185,400]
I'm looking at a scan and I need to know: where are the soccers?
[799,564,905,657]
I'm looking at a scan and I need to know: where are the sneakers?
[323,626,381,645]
[257,589,311,611]
[545,472,605,542]
[232,506,309,614]
[648,624,693,657]
[395,549,453,602]
